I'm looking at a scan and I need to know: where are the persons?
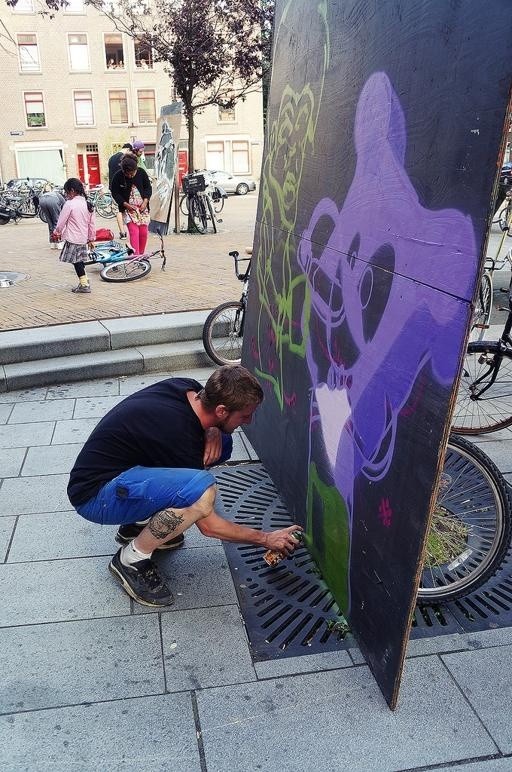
[108,143,133,239]
[132,140,157,182]
[52,177,95,293]
[111,154,152,255]
[108,58,124,70]
[39,191,67,250]
[66,364,304,607]
[140,59,148,70]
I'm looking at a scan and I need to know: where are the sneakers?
[115,524,186,550]
[108,545,174,607]
[49,240,65,250]
[72,279,92,295]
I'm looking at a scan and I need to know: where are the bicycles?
[179,169,228,235]
[450,188,512,438]
[0,176,119,226]
[81,228,167,285]
[202,247,255,366]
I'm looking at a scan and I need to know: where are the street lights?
[128,123,139,143]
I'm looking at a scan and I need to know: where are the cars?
[499,161,512,185]
[210,169,257,195]
